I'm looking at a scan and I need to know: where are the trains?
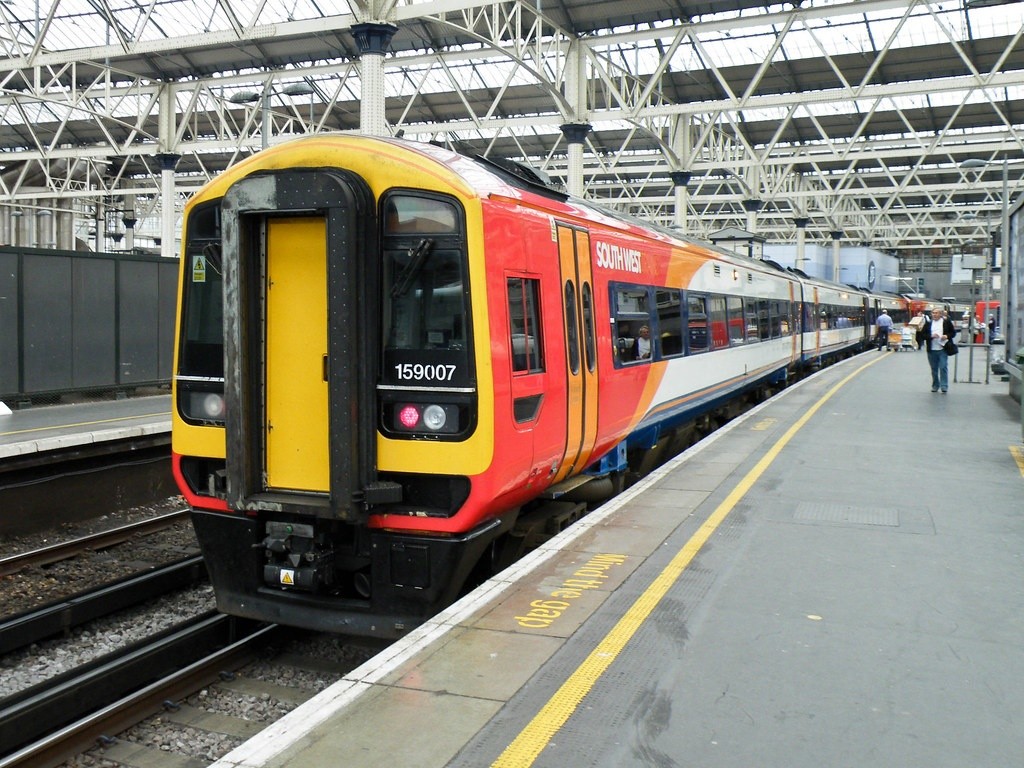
[172,132,977,643]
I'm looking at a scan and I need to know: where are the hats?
[821,312,826,316]
[882,310,887,313]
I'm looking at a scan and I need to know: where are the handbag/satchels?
[943,341,959,356]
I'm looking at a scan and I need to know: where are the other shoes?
[942,388,947,392]
[887,349,890,351]
[878,349,881,351]
[931,389,937,392]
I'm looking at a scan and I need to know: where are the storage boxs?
[901,316,925,345]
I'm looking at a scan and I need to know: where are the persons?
[876,310,893,351]
[970,315,980,344]
[988,314,995,345]
[636,326,650,360]
[916,307,959,393]
